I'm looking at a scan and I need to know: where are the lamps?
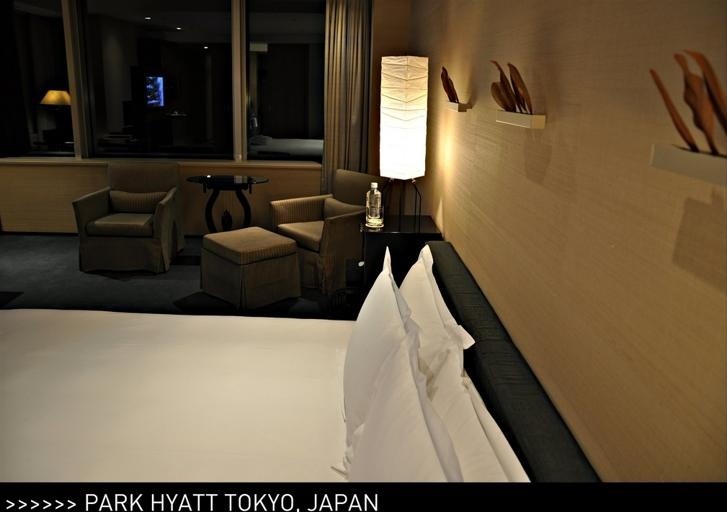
[39,90,71,106]
[379,54,432,234]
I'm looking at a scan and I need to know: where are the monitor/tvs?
[144,75,165,108]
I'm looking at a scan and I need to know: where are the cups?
[365,206,384,226]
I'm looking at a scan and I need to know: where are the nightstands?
[358,213,443,306]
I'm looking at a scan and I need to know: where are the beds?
[249,135,324,165]
[0,240,607,483]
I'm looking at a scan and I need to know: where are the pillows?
[322,195,366,222]
[108,188,168,215]
[331,242,535,483]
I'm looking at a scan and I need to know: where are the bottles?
[366,183,381,218]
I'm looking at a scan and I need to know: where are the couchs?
[198,224,303,309]
[268,167,387,296]
[70,156,189,275]
